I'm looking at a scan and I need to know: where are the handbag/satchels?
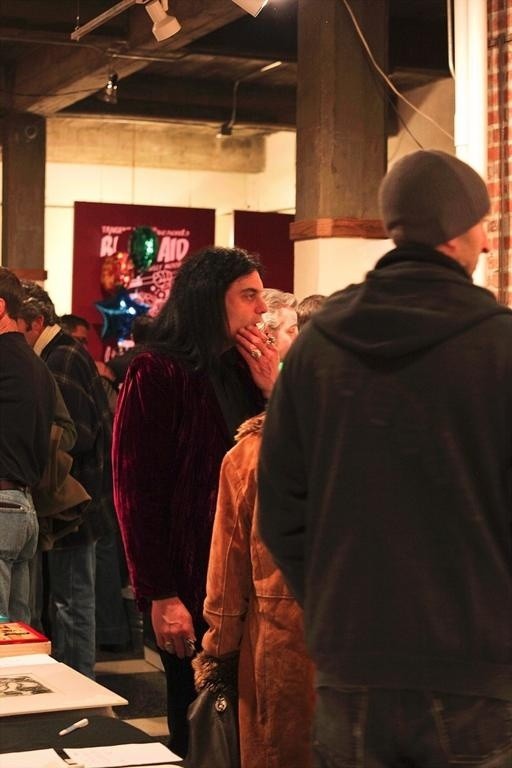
[188,656,240,768]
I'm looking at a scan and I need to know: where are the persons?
[256,149,510,767]
[2,268,158,682]
[112,244,327,768]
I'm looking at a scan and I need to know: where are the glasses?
[72,335,88,346]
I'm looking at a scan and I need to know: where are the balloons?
[127,225,163,272]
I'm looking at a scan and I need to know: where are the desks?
[0,715,184,768]
[0,655,129,717]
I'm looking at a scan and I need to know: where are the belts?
[0,479,31,493]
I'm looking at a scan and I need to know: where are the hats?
[379,150,491,248]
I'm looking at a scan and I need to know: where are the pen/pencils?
[59,717,88,737]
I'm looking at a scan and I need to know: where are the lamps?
[216,59,282,139]
[104,54,176,105]
[70,0,270,43]
